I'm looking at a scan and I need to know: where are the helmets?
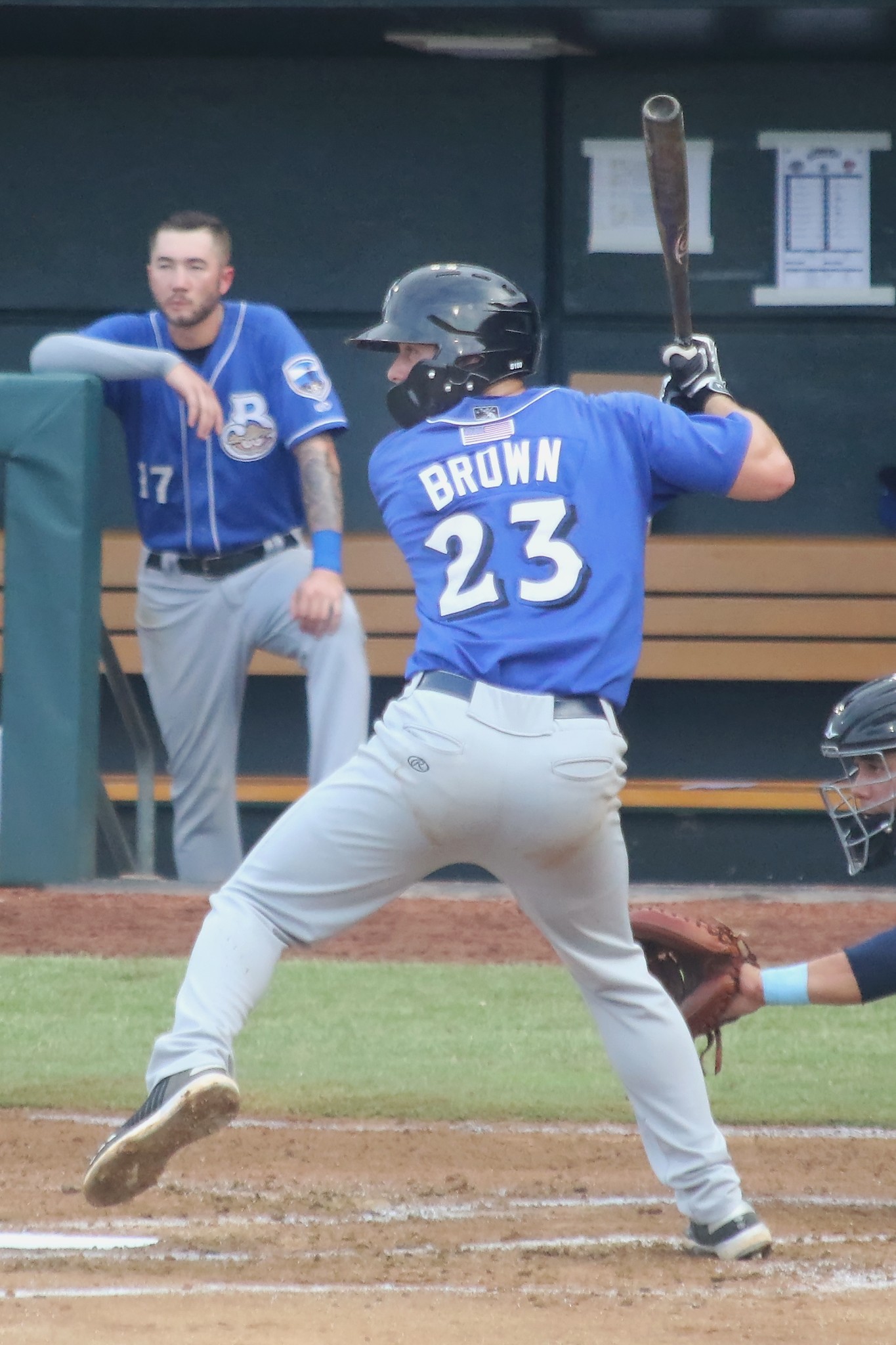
[820,674,896,759]
[348,260,540,430]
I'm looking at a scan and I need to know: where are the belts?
[417,669,605,721]
[145,535,295,581]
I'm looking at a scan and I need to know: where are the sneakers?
[691,1212,772,1261]
[84,1066,240,1207]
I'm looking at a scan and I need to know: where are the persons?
[81,262,797,1261]
[29,206,373,888]
[625,674,896,1039]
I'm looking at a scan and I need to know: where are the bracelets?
[761,962,811,1007]
[311,529,342,573]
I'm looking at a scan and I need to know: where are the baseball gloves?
[630,905,765,1039]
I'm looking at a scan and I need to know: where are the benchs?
[100,527,896,811]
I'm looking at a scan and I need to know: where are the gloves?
[662,334,732,411]
[660,376,693,415]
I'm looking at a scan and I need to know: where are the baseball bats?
[641,95,694,347]
